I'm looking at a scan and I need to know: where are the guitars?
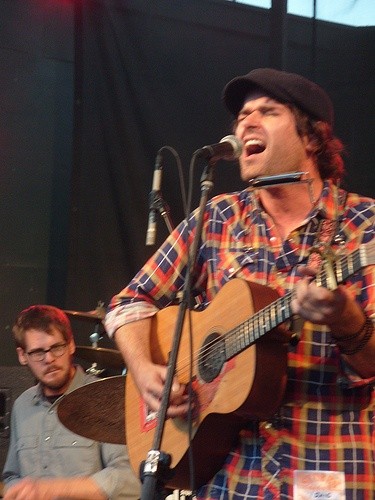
[124,234,375,492]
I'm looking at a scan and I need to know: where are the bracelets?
[328,314,374,355]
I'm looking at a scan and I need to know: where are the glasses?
[25,341,69,362]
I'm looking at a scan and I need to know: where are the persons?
[102,68,375,500]
[1,305,143,500]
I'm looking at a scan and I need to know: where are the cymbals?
[65,309,108,323]
[73,346,125,369]
[57,374,127,446]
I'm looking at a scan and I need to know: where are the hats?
[223,68,335,124]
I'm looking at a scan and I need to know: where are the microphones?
[146,155,163,245]
[193,135,243,162]
